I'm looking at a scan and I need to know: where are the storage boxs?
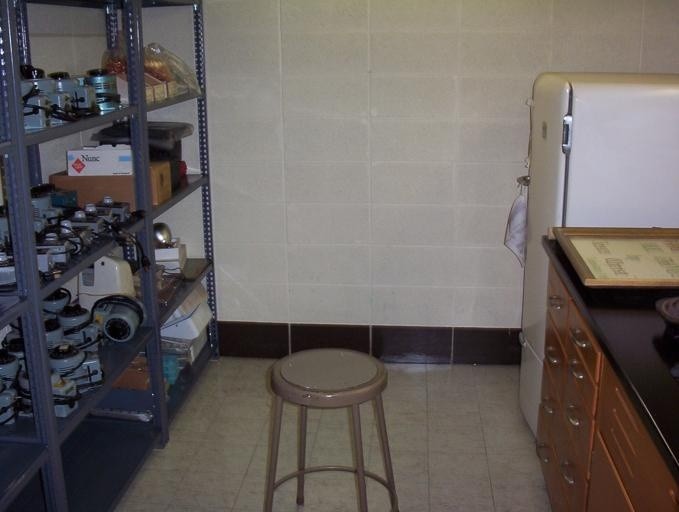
[49,143,172,213]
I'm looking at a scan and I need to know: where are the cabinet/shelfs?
[1,0,221,511]
[536,259,679,512]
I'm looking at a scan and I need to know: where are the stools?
[264,348,398,512]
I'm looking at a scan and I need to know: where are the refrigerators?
[515,70,679,442]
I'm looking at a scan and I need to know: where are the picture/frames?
[551,227,678,290]
